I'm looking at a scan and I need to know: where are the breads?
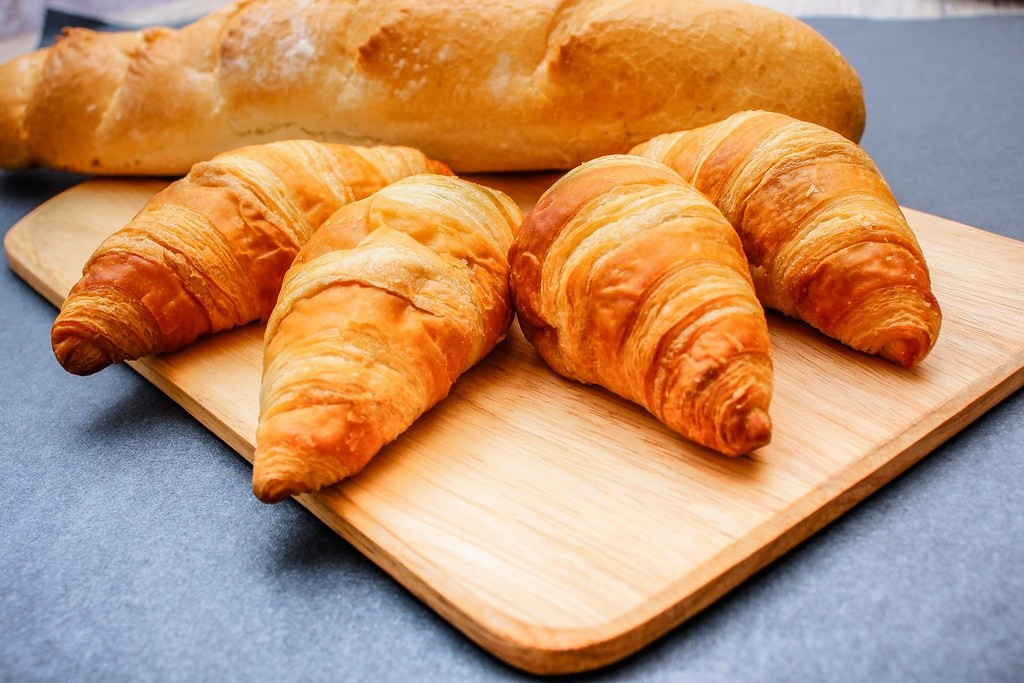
[51,137,460,377]
[251,172,525,503]
[622,110,944,370]
[506,155,773,457]
[0,0,865,177]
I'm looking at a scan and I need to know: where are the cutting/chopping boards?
[4,147,1024,676]
